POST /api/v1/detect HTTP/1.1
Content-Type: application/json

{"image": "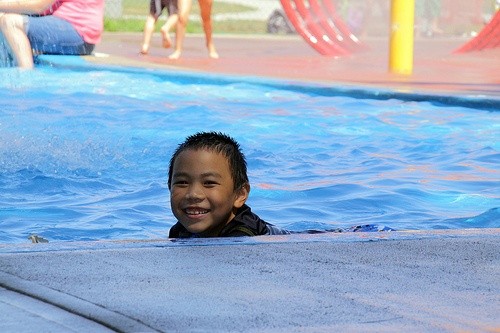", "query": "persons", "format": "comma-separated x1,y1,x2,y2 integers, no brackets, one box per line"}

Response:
0,0,218,69
167,131,394,238
